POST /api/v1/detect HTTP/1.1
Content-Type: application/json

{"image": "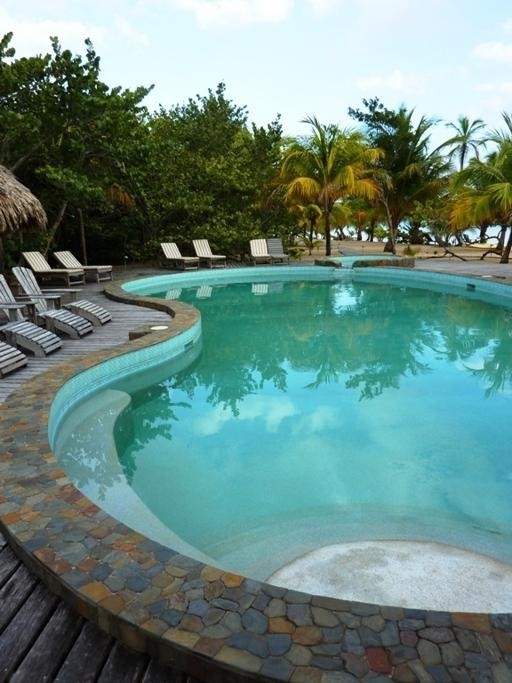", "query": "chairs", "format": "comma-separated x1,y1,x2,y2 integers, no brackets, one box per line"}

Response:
161,238,227,271
21,250,114,286
0,267,113,375
249,238,290,265
